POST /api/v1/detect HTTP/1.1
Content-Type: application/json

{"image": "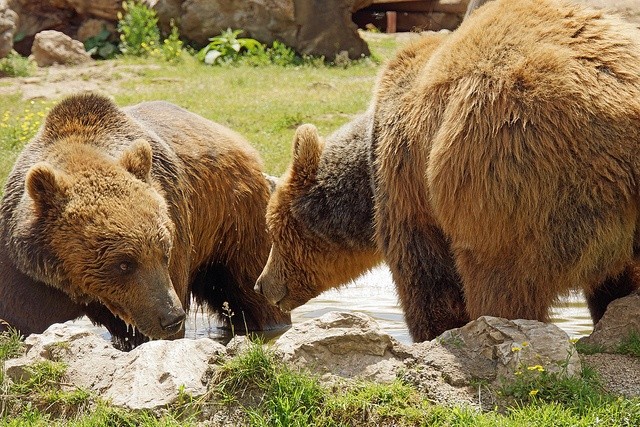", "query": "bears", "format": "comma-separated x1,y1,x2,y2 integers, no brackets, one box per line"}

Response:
253,0,640,343
0,95,291,348
